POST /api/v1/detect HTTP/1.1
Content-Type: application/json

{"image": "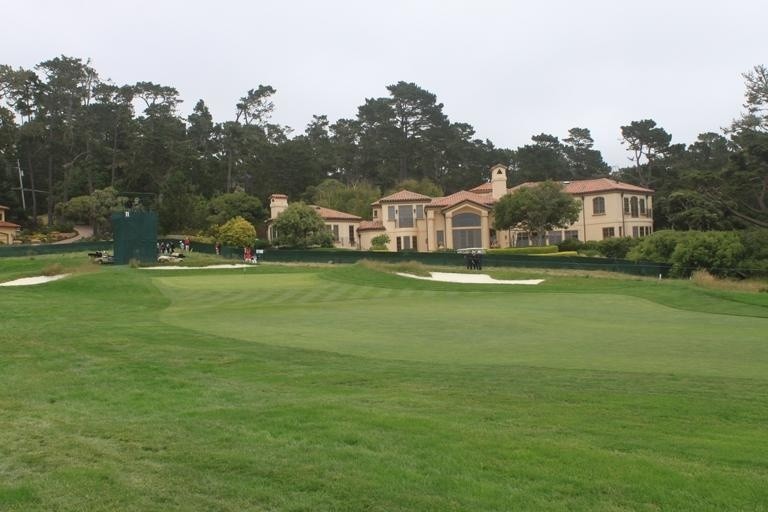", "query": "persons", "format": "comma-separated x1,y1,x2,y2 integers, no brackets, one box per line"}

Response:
466,250,482,270
157,237,190,255
243,247,258,264
215,242,220,254
